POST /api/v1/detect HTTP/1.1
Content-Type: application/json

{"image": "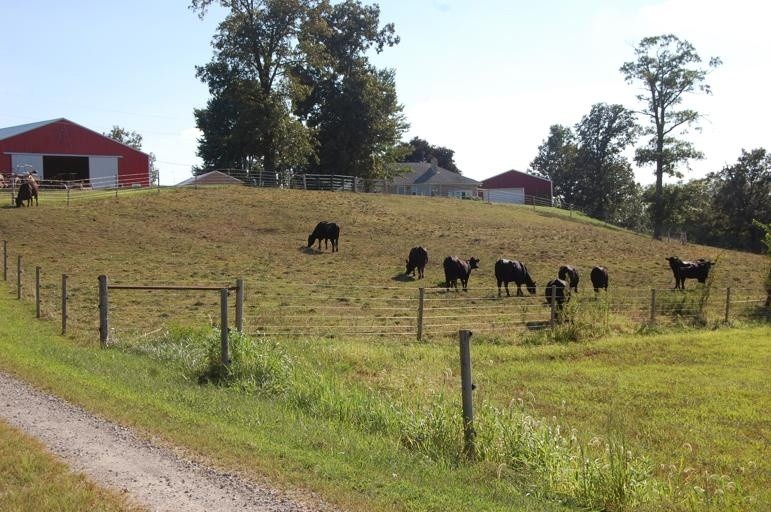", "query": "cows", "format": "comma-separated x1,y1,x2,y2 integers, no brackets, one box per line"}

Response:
665,254,716,289
443,255,480,292
405,246,429,279
545,278,571,322
558,264,579,293
590,265,609,301
14,182,39,207
0,172,35,188
495,258,536,297
307,220,340,253
